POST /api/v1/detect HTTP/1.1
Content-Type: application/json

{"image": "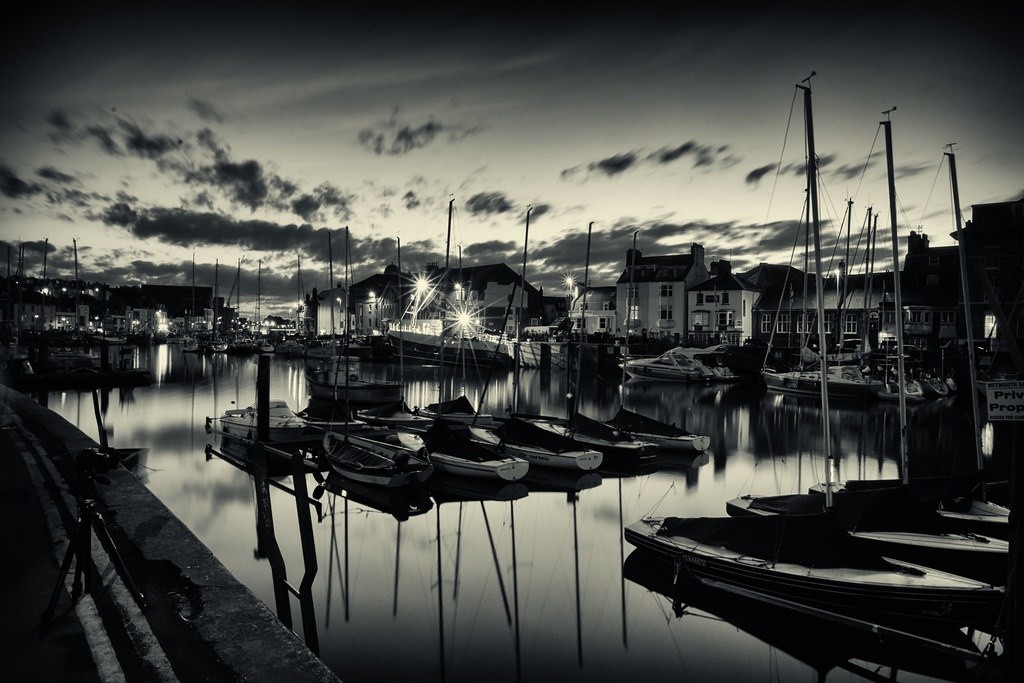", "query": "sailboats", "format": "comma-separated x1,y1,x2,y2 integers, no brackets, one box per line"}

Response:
1,70,1024,633
